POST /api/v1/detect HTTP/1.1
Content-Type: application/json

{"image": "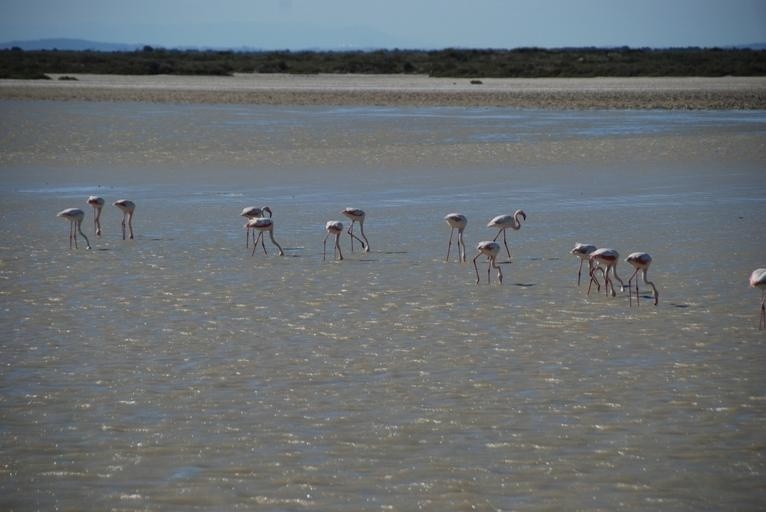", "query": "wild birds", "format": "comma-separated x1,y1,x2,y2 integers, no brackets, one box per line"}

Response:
568,242,659,308
473,241,503,284
112,199,136,240
85,195,104,236
487,209,526,258
749,268,766,330
56,208,91,250
339,207,369,252
323,220,343,261
444,213,467,264
239,204,284,256
